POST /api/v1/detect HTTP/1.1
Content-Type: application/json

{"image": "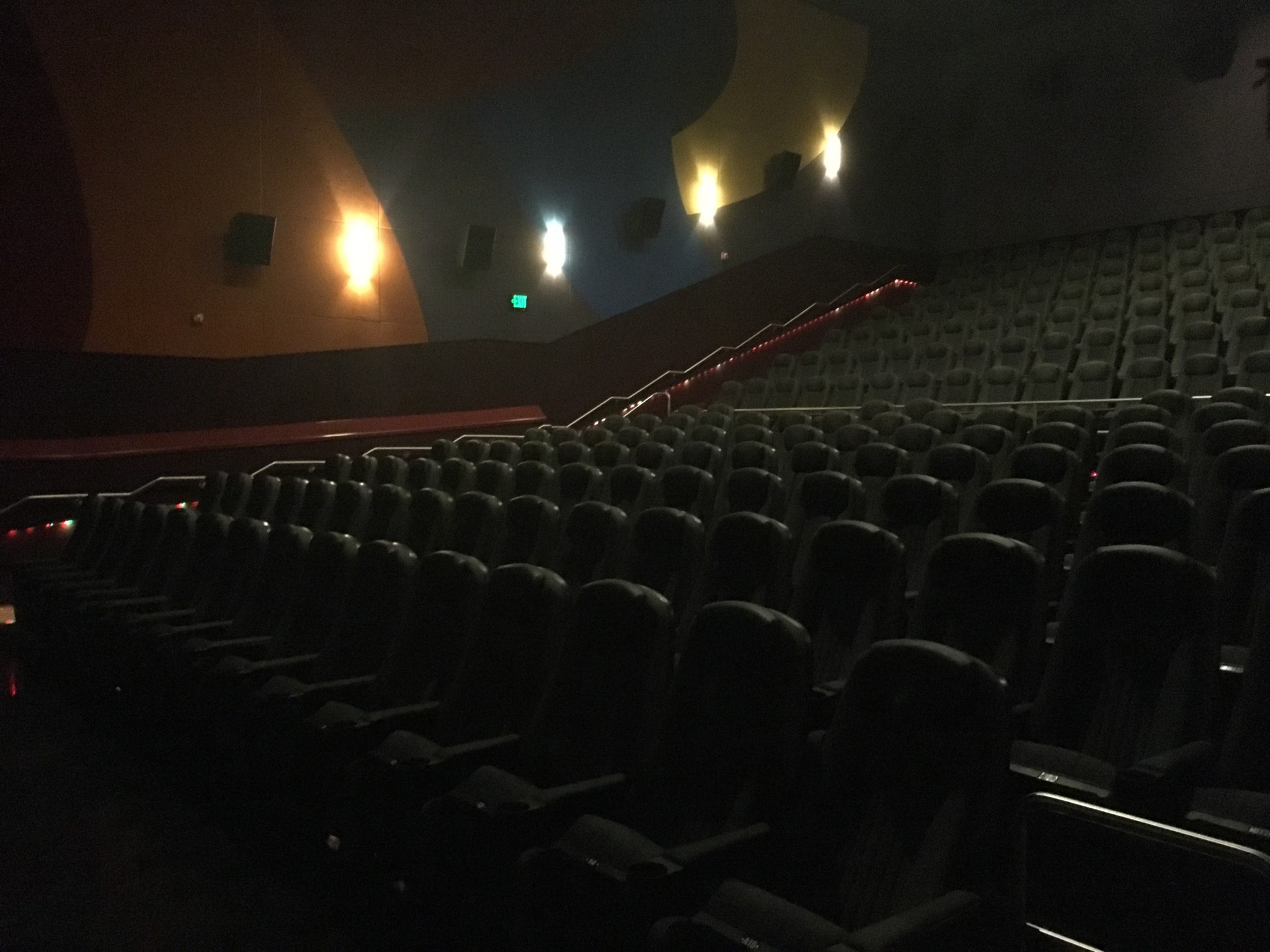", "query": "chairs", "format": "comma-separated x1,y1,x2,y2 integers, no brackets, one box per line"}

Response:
0,204,1270,952
